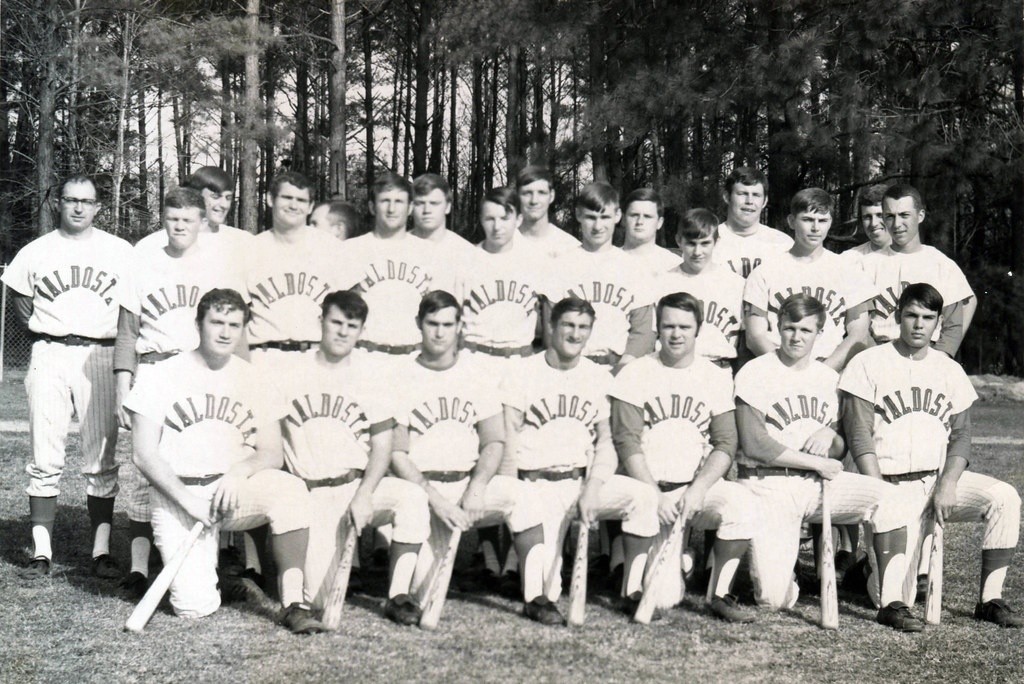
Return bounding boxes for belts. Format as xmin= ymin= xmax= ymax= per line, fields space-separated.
xmin=357 ymin=340 xmax=423 ymax=356
xmin=459 ymin=341 xmax=539 ymax=359
xmin=180 ymin=474 xmax=224 ymax=486
xmin=38 ymin=333 xmax=116 ymax=348
xmin=517 ymin=468 xmax=586 ymax=481
xmin=587 ymin=354 xmax=621 ymax=365
xmin=883 ymin=468 xmax=940 ymax=485
xmin=423 ymin=470 xmax=470 ymax=483
xmin=301 ymin=470 xmax=366 ymax=490
xmin=248 ymin=340 xmax=311 ymax=353
xmin=737 ymin=466 xmax=814 ymax=480
xmin=138 ymin=352 xmax=173 ymax=364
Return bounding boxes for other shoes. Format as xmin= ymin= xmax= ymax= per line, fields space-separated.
xmin=878 ymin=600 xmax=921 ymax=631
xmin=708 ymin=594 xmax=754 ymax=622
xmin=281 ymin=601 xmax=320 ymax=634
xmin=385 ymin=594 xmax=420 ymax=626
xmin=92 ymin=554 xmax=121 ymax=580
xmin=974 ymin=599 xmax=1023 ymax=628
xmin=20 ymin=555 xmax=52 ymax=579
xmin=525 ymin=595 xmax=565 ymax=626
xmin=627 ymin=596 xmax=663 ymax=625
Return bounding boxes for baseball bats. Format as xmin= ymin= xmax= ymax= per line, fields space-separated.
xmin=566 ymin=520 xmax=589 ymax=635
xmin=317 ymin=523 xmax=358 ymax=631
xmin=417 ymin=527 xmax=462 ymax=633
xmin=632 ymin=514 xmax=687 ymax=626
xmin=124 ymin=520 xmax=204 ymax=633
xmin=820 ymin=479 xmax=839 ymax=631
xmin=924 ymin=521 xmax=943 ymax=626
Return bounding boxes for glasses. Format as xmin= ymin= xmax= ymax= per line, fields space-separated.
xmin=59 ymin=196 xmax=99 ymax=206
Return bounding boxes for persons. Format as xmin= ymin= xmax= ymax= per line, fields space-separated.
xmin=274 ymin=290 xmax=429 ymax=628
xmin=123 ymin=289 xmax=322 ymax=632
xmin=2 ymin=165 xmax=1020 ymax=634
